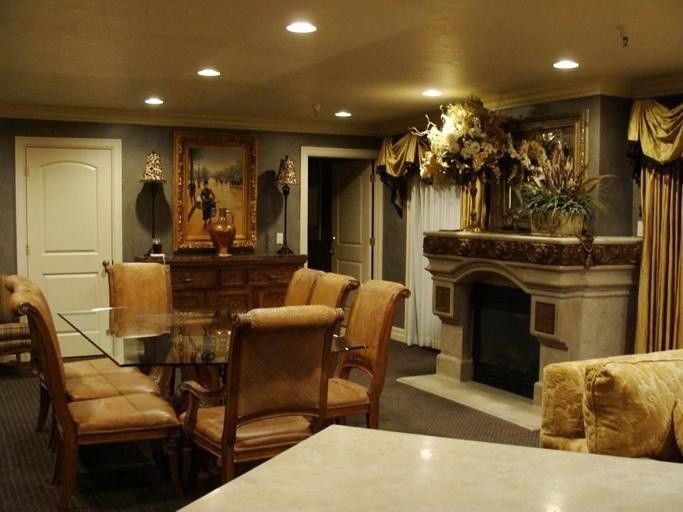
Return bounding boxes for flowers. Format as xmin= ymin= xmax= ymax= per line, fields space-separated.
xmin=507 ymin=133 xmax=618 ymax=242
xmin=408 ymin=94 xmax=510 ymax=186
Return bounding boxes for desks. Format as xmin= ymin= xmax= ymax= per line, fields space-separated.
xmin=175 ymin=424 xmax=683 ymax=512
xmin=56 ymin=309 xmax=371 ymax=409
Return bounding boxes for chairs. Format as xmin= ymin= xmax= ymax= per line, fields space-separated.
xmin=314 ymin=279 xmax=411 ymax=430
xmin=179 ymin=304 xmax=345 ymax=485
xmin=103 ymin=262 xmax=174 ymax=307
xmin=106 ymin=310 xmax=172 ymax=340
xmin=282 ymin=268 xmax=324 ymax=306
xmin=4 ymin=274 xmax=140 ymax=431
xmin=2 ymin=292 xmax=182 ymax=510
xmin=307 ymin=272 xmax=361 ymax=338
xmin=12 ymin=279 xmax=161 ymax=484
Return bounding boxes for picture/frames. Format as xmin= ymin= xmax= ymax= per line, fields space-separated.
xmin=500 ymin=109 xmax=589 ymax=222
xmin=172 ymin=127 xmax=258 ymax=252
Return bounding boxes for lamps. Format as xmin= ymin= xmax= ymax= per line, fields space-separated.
xmin=274 ymin=155 xmax=299 ymax=254
xmin=140 ymin=151 xmax=167 ymax=256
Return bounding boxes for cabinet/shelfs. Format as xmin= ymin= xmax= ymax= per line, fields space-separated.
xmin=171 ymin=266 xmax=300 ymax=312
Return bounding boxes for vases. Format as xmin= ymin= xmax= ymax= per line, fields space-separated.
xmin=529 ymin=209 xmax=584 ymax=238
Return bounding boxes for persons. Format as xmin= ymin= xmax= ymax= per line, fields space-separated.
xmin=188 ymin=173 xmax=243 ymax=227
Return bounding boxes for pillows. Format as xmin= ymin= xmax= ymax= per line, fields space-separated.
xmin=582 ymin=356 xmax=683 ymax=461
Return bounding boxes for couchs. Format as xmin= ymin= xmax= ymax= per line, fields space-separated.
xmin=539 ymin=349 xmax=683 ymax=461
